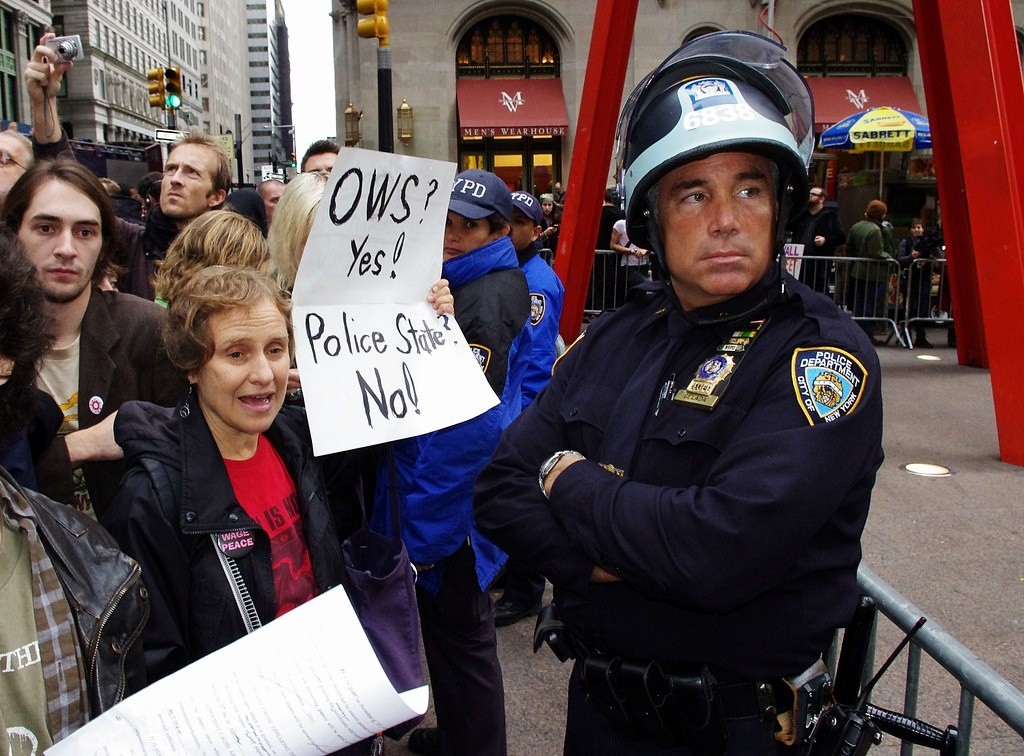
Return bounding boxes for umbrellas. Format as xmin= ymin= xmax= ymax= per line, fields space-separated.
xmin=818 ymin=106 xmax=932 ymax=201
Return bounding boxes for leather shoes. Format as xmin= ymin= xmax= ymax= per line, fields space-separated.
xmin=491 ymin=594 xmax=543 ymax=628
xmin=407 ymin=726 xmax=447 ymax=756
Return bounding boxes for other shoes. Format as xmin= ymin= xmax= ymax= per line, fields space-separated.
xmin=871 ymin=336 xmax=887 ymax=347
xmin=913 ymin=337 xmax=933 ymax=349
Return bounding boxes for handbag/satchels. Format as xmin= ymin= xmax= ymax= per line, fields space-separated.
xmin=340 ymin=523 xmax=425 ymax=693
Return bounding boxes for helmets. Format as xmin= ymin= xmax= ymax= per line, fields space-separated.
xmin=612 ymin=53 xmax=811 ymax=251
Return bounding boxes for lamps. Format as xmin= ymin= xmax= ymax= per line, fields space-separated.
xmin=344 ymin=102 xmax=360 ymax=149
xmin=396 ymin=97 xmax=414 ymax=146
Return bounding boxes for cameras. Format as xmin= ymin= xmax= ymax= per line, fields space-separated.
xmin=45 ymin=35 xmax=85 ymax=63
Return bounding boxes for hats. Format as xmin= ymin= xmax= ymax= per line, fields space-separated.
xmin=224 ymin=187 xmax=268 ymax=237
xmin=510 ymin=190 xmax=544 ymax=225
xmin=449 ymin=168 xmax=513 ymax=222
xmin=539 ymin=193 xmax=555 ymax=203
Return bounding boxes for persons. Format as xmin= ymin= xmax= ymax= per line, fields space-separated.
xmin=470 ymin=30 xmax=885 ymax=756
xmin=584 ymin=189 xmax=657 ymax=324
xmin=0 ymin=33 xmax=565 ymax=756
xmin=788 ymin=188 xmax=955 ymax=347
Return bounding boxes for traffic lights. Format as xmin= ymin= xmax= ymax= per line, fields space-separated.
xmin=147 ymin=67 xmax=166 ymax=110
xmin=290 ymin=153 xmax=296 ymax=168
xmin=357 ymin=0 xmax=389 ymax=40
xmin=165 ymin=66 xmax=182 ymax=111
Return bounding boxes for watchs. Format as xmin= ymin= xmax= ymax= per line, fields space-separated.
xmin=538 ymin=449 xmax=584 ymax=497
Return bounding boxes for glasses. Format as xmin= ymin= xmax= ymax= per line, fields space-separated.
xmin=0 ymin=151 xmax=28 ymax=171
xmin=809 ymin=192 xmax=821 ymax=198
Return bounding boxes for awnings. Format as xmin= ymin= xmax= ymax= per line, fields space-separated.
xmin=803 ymin=75 xmax=922 ymax=133
xmin=457 ymin=78 xmax=568 ymax=137
